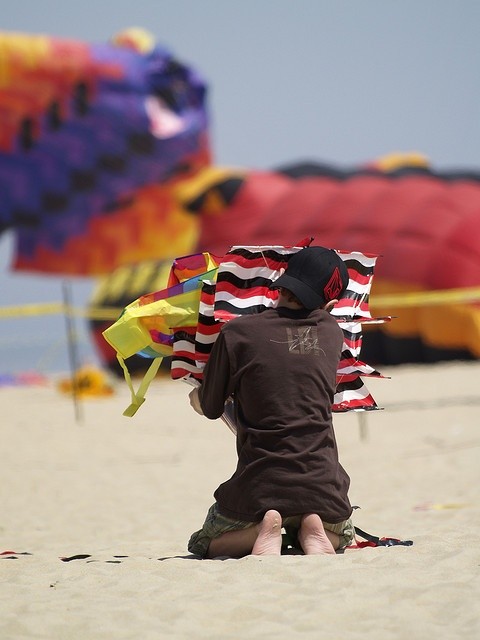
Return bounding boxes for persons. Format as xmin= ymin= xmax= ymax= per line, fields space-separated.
xmin=188 ymin=246 xmax=356 ymax=556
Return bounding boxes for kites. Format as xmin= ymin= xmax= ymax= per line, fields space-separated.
xmin=101 ymin=237 xmax=397 ymax=417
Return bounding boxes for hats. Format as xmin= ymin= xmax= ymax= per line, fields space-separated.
xmin=269 ymin=245 xmax=348 ymax=310
